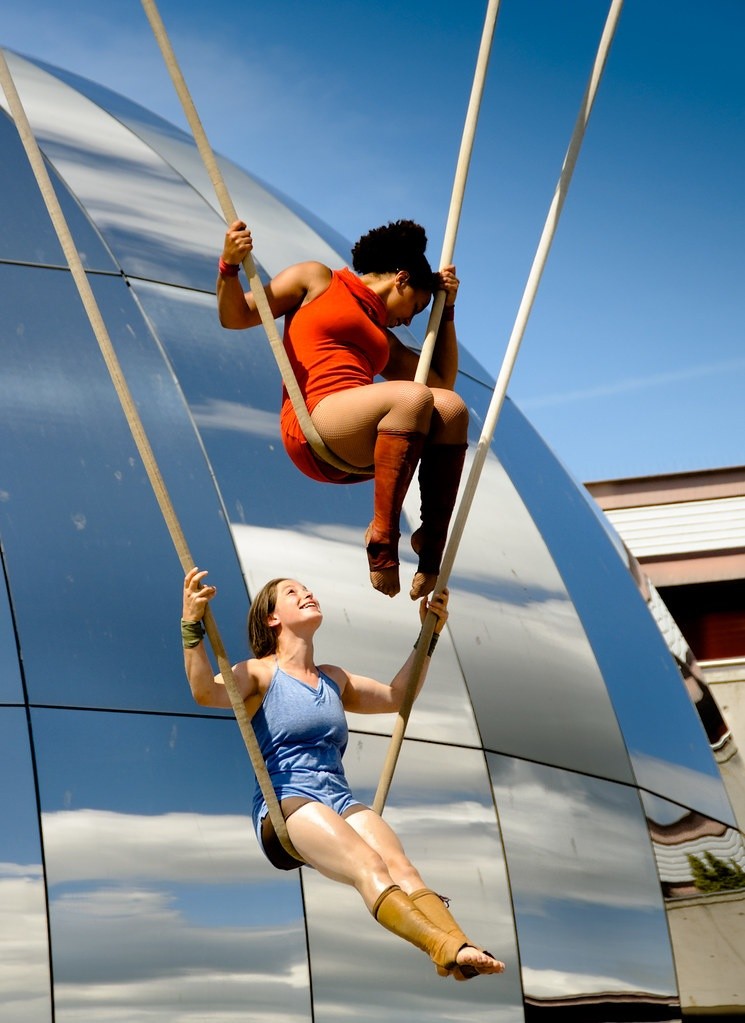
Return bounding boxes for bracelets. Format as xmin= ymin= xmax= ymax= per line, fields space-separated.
xmin=181 ymin=619 xmax=206 ymax=650
xmin=442 ymin=306 xmax=456 ymax=320
xmin=219 ymin=259 xmax=241 ymax=278
xmin=414 ymin=628 xmax=438 ymax=658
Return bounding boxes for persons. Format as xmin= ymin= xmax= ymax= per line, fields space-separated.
xmin=180 ymin=566 xmax=507 ymax=981
xmin=216 ymin=219 xmax=471 ymax=599
